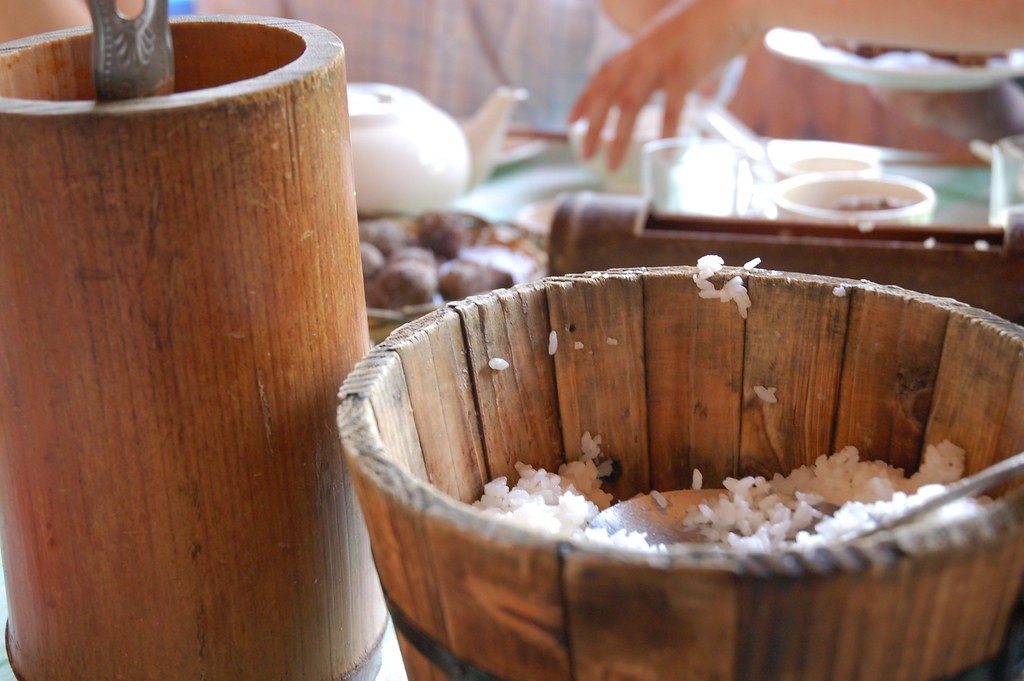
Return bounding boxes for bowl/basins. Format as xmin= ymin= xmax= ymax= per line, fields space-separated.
xmin=571 ymin=107 xmax=706 ymax=189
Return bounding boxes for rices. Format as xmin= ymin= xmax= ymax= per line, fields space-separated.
xmin=462 ymin=255 xmax=1008 ymax=552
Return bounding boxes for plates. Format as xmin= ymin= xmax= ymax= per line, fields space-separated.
xmin=764 ymin=26 xmax=1024 ymax=92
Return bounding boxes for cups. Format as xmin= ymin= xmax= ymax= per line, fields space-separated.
xmin=774 ymin=172 xmax=937 ymax=234
xmin=987 ymin=134 xmax=1024 ymax=229
xmin=641 ymin=136 xmax=883 ymax=224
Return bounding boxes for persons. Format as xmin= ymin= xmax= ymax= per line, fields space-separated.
xmin=567 ymin=0 xmax=1024 ymax=170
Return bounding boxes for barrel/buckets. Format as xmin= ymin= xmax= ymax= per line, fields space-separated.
xmin=334 ymin=265 xmax=1024 ymax=681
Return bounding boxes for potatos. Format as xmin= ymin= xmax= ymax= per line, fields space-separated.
xmin=355 ymin=210 xmax=525 ymax=309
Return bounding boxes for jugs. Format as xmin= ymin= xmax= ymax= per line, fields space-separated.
xmin=346 ymin=76 xmax=526 ymax=216
